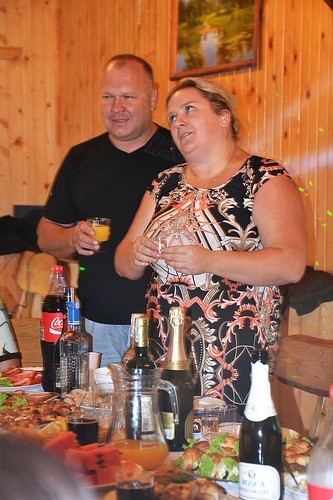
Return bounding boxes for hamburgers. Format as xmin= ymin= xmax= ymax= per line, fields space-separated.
xmin=173 ymin=434 xmax=314 ymax=483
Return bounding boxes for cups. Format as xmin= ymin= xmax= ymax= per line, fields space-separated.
xmin=88 ymin=352 xmax=102 ymax=389
xmin=86 ymin=217 xmax=111 ymax=255
xmin=117 ymin=471 xmax=155 ymax=500
xmin=64 ymin=410 xmax=98 ymax=445
xmin=153 ymin=237 xmax=168 ymax=259
xmin=202 ymin=403 xmax=238 ymax=442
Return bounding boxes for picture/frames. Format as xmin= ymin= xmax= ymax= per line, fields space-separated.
xmin=168 ymin=0 xmax=262 ymax=82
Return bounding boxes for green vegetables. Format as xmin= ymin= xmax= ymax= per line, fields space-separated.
xmin=0 ymin=372 xmax=28 ymax=413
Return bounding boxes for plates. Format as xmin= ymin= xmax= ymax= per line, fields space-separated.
xmin=0 ymin=366 xmax=123 ymax=430
xmin=83 ymin=460 xmax=143 ymax=491
xmin=170 ymin=450 xmax=239 ymax=488
xmin=217 ymin=424 xmax=300 ymax=442
xmin=192 ymin=395 xmax=226 ymax=411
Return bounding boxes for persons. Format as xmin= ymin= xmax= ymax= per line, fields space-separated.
xmin=0 ymin=295 xmax=24 ymax=372
xmin=113 ymin=76 xmax=305 ymax=417
xmin=35 ymin=54 xmax=187 ymax=368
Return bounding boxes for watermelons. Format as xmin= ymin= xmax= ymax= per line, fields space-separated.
xmin=41 ymin=430 xmax=135 ymax=486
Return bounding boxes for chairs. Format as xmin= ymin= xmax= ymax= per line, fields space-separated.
xmin=274 ymin=266 xmax=333 ymax=440
xmin=8 ymin=248 xmax=59 ymax=368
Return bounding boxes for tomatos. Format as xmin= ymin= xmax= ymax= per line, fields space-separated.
xmin=4 ymin=367 xmax=42 ymax=387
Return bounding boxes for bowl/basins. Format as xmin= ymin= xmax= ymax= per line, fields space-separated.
xmin=92 ymin=367 xmax=114 ymax=392
xmin=285 ymin=474 xmax=308 ymax=500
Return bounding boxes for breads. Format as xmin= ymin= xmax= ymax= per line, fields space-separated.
xmin=100 ymin=468 xmax=231 ymax=500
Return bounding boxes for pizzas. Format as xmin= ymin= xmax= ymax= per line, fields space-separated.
xmin=0 ymin=399 xmax=80 ymax=432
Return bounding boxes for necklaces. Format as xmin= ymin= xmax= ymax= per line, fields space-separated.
xmin=190 ymin=148 xmax=234 ymax=181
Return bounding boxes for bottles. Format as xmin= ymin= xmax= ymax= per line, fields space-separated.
xmin=125 ymin=317 xmax=157 ymax=440
xmin=306 ymin=383 xmax=333 ymax=500
xmin=159 ymin=306 xmax=194 ymax=453
xmin=239 ymin=348 xmax=284 ymax=500
xmin=40 ymin=265 xmax=89 ymax=400
xmin=120 ymin=313 xmax=155 ymax=369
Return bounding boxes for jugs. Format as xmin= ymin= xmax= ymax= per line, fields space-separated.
xmin=104 ymin=362 xmax=180 ymax=471
